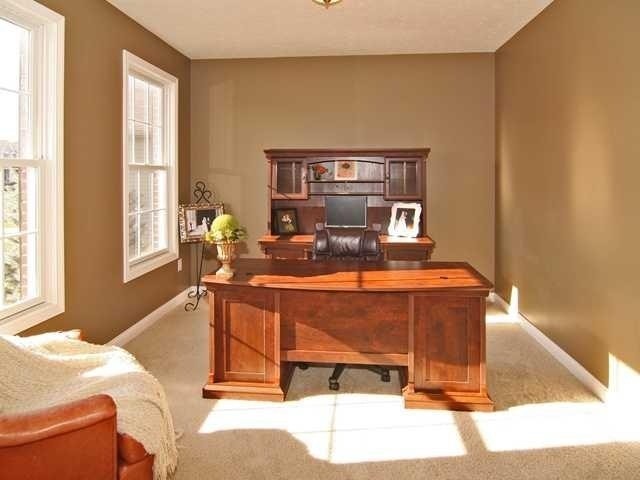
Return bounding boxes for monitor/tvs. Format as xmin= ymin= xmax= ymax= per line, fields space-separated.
xmin=325 ymin=195 xmax=367 ymax=228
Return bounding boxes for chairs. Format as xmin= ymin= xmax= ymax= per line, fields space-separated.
xmin=0 ymin=332 xmax=180 ymax=479
xmin=298 ymin=228 xmax=389 ymax=391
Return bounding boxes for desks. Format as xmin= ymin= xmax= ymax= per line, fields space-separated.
xmin=200 ymin=258 xmax=496 ymax=412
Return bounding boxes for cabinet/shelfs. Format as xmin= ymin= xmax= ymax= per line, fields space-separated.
xmin=265 ymin=148 xmax=436 ymax=263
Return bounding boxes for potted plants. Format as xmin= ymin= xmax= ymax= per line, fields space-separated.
xmin=206 ymin=214 xmax=248 ymax=281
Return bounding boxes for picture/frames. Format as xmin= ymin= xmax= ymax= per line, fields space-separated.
xmin=179 ymin=204 xmax=223 ymax=243
xmin=389 ymin=203 xmax=422 ymax=237
xmin=335 ymin=161 xmax=358 ymax=180
xmin=271 ymin=208 xmax=299 ymax=235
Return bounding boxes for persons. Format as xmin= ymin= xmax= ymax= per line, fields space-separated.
xmin=397 ymin=211 xmax=413 ymax=232
xmin=197 ymin=216 xmax=212 ymax=232
xmin=282 ymin=213 xmax=296 ymax=232
